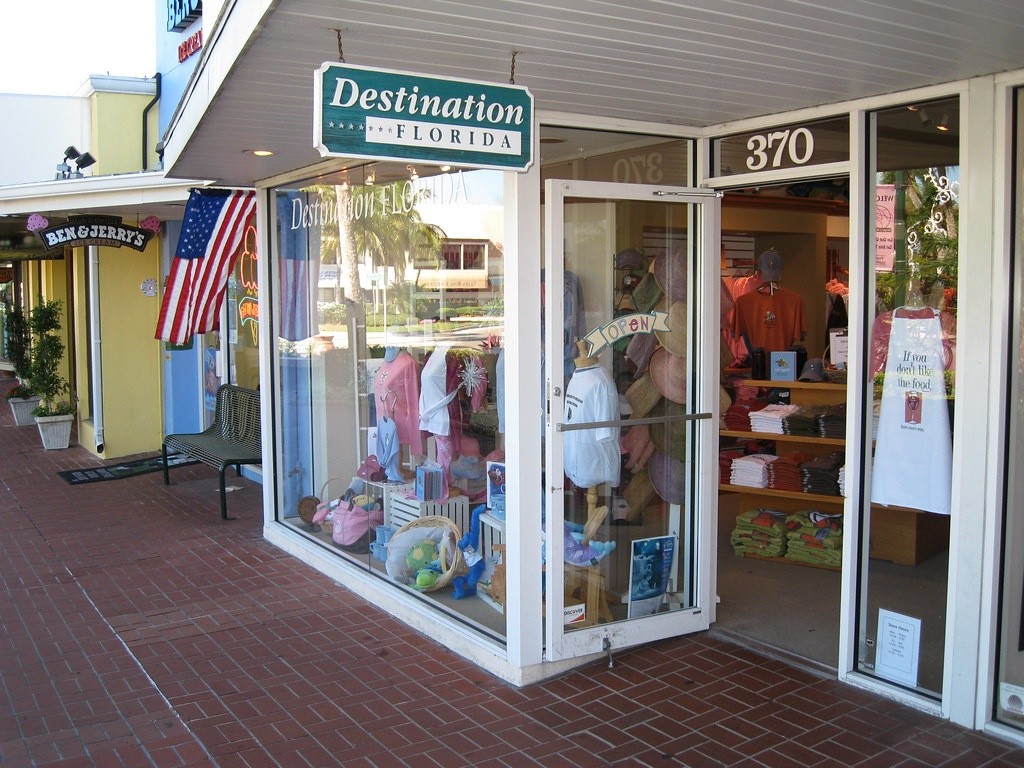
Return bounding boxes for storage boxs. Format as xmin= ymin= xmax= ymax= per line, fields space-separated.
xmin=829 ymin=328 xmax=848 ymax=365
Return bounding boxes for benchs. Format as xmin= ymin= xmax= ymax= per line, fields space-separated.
xmin=162 ymin=384 xmax=262 ymax=521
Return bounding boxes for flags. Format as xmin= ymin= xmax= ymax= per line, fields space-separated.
xmin=154 ymin=188 xmax=258 ymax=346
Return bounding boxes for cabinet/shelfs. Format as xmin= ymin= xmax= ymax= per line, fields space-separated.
xmin=719 ymin=379 xmax=955 ymax=571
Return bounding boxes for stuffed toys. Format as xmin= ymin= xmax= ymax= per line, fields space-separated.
xmin=452 ymin=505 xmax=486 ymax=599
xmin=406 ymin=539 xmax=450 ymax=588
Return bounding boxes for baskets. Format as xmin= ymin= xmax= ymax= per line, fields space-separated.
xmin=318 ymin=478 xmax=348 ymax=536
xmin=822 ymin=345 xmax=847 ymax=383
xmin=385 ymin=516 xmax=463 ymax=592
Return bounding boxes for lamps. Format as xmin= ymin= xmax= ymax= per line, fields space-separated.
xmin=406 ymin=164 xmax=420 ymax=181
xmin=364 ymin=169 xmax=376 ymax=185
xmin=69 ymin=152 xmax=96 ymax=178
xmin=56 ymin=146 xmax=81 ymax=179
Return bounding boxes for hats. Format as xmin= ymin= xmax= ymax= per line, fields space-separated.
xmin=798 ymin=358 xmax=829 ymax=381
xmin=758 ymin=250 xmax=783 ymax=281
xmin=449 ymin=454 xmax=483 ymax=479
xmin=616 ymin=248 xmax=687 ymax=503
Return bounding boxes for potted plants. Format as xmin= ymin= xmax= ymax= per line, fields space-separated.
xmin=27 ymin=291 xmax=75 ymax=449
xmin=0 ymin=281 xmax=43 ymax=427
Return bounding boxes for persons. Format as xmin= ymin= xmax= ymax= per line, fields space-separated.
xmin=563 ymin=353 xmax=621 ymax=489
xmin=374 ymin=345 xmax=424 ymax=456
xmin=418 ymin=339 xmax=463 ymax=440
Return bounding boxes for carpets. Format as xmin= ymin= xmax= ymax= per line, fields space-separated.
xmin=57 ymin=451 xmax=201 ymax=485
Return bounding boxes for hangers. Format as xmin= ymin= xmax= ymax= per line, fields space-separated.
xmin=825 ymin=267 xmax=849 ymax=288
xmin=757 ymin=284 xmax=778 ymax=292
xmin=731 ymin=260 xmax=761 ymax=277
